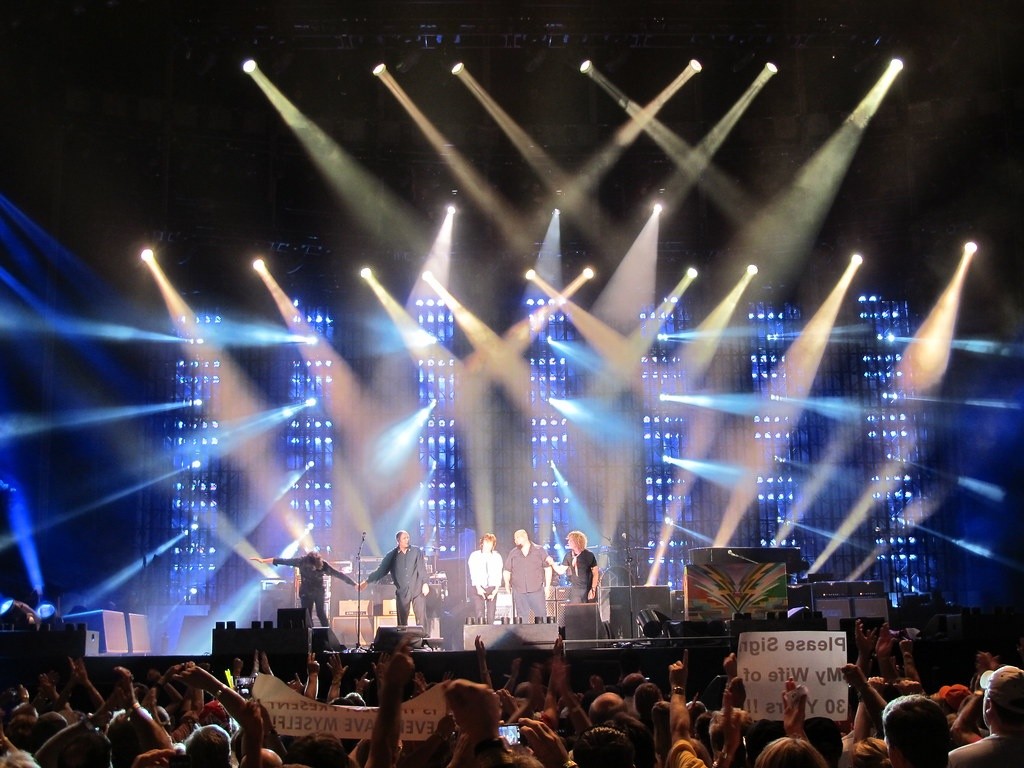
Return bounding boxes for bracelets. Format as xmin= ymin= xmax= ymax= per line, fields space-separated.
xmin=592 ymin=586 xmax=597 ymax=598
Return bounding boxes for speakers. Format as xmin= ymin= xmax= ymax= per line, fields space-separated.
xmin=632 ymin=586 xmax=670 ymax=638
xmin=597 ymin=586 xmax=632 ymax=640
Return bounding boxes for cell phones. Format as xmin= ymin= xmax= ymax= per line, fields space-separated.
xmin=235 ymin=676 xmax=256 ymax=689
xmin=498 ymin=723 xmax=527 ymax=748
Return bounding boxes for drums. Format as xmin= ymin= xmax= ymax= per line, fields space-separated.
xmin=600 ymin=566 xmax=634 ymax=586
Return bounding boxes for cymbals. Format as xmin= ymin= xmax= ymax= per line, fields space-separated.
xmin=625 ymin=547 xmax=651 ymax=550
xmin=587 ymin=546 xmax=612 ymax=549
xmin=598 ymin=550 xmax=618 ymax=553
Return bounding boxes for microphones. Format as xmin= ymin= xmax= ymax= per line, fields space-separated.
xmin=358 ymin=531 xmax=367 ymax=555
xmin=622 ymin=532 xmax=628 ymax=540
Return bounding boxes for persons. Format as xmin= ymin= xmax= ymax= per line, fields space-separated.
xmin=548 ymin=530 xmax=600 ymax=604
xmin=0 ymin=615 xmax=1024 ymax=768
xmin=469 ymin=533 xmax=504 ymax=623
xmin=503 ymin=527 xmax=554 ymax=624
xmin=357 ymin=530 xmax=431 ymax=638
xmin=248 ymin=551 xmax=360 ymax=628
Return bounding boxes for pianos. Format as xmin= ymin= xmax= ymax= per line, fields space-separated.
xmin=687 ymin=547 xmax=808 ymax=585
